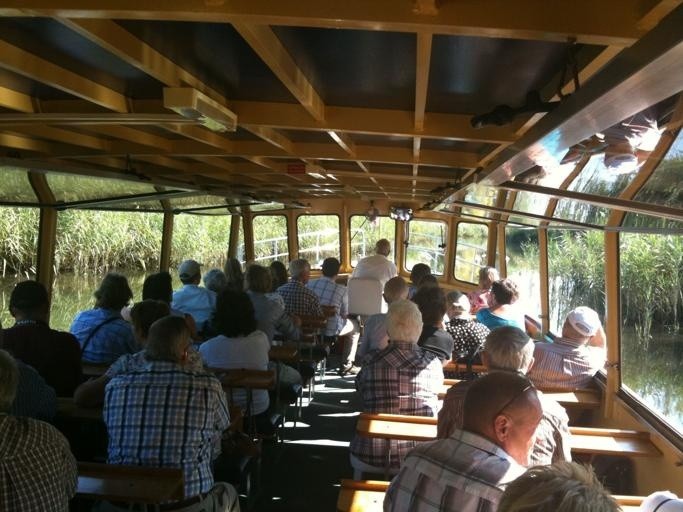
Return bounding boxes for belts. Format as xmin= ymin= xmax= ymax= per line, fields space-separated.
xmin=110 ymin=494 xmax=207 ymax=512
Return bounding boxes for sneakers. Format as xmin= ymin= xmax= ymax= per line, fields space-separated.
xmin=340 ymin=363 xmax=361 ymax=375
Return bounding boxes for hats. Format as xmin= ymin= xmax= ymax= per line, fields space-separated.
xmin=179 ymin=260 xmax=204 ymax=280
xmin=567 ymin=306 xmax=599 ymax=336
xmin=12 ymin=281 xmax=47 ymax=308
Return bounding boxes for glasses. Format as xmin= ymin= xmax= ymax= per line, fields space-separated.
xmin=493 ymin=379 xmax=536 ymax=434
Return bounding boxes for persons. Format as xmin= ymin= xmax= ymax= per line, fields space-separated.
xmin=0 ymin=240 xmax=622 ymax=511
